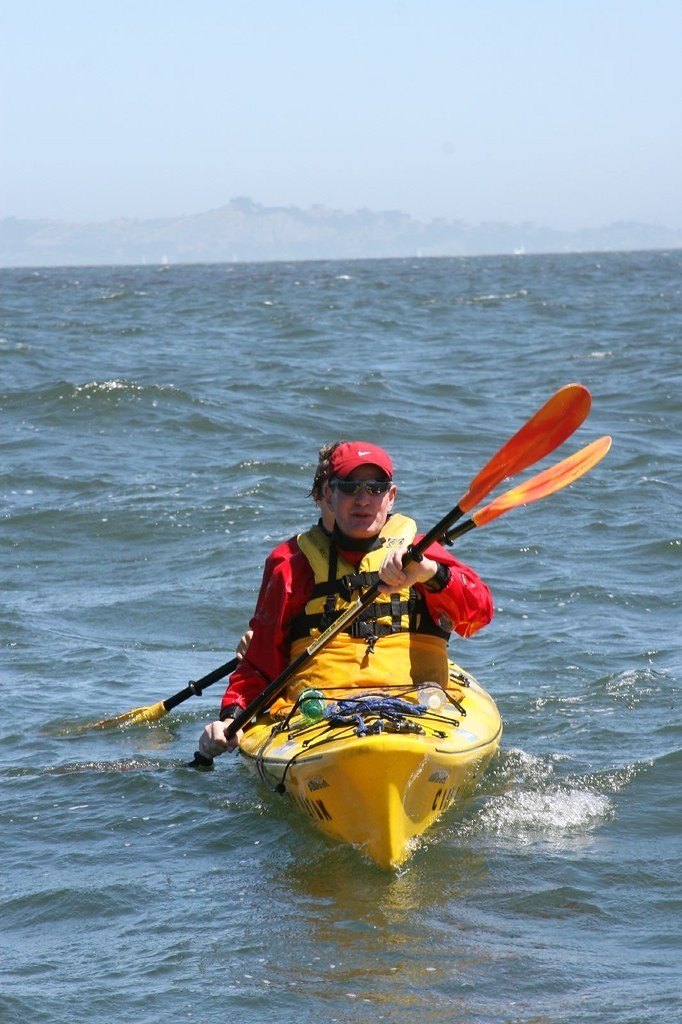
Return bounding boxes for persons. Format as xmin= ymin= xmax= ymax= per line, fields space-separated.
xmin=198 ymin=439 xmax=495 ymax=759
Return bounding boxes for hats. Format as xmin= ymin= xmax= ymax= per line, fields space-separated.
xmin=329 ymin=440 xmax=392 ymax=480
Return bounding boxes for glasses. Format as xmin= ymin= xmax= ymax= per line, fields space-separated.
xmin=330 ymin=478 xmax=388 ymax=496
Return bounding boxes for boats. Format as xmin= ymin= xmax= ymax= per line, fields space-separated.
xmin=236 ymin=655 xmax=504 ymax=872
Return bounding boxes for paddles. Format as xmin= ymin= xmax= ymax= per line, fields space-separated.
xmin=174 ymin=381 xmax=593 ymax=772
xmin=92 ymin=433 xmax=614 ymax=727
